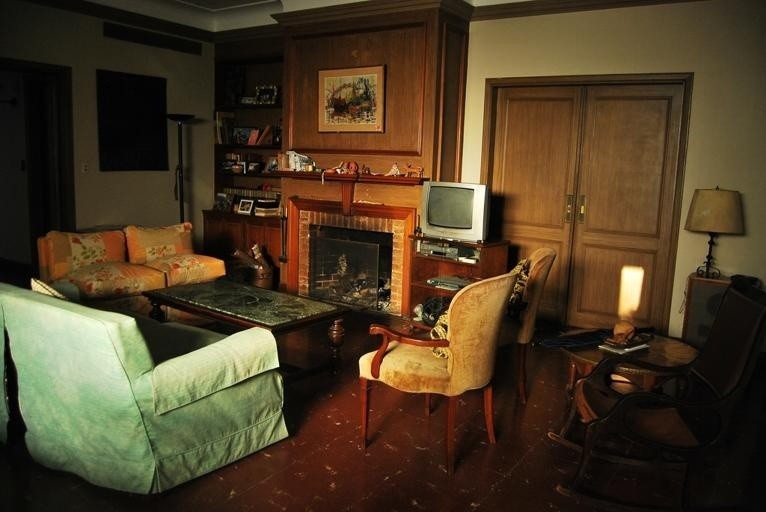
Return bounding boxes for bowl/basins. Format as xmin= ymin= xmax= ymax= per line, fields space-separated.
xmin=231 ymin=164 xmax=243 ymax=175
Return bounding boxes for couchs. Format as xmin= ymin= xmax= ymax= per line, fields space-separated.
xmin=37 ymin=225 xmax=216 ymax=326
xmin=0 ymin=283 xmax=290 ymax=495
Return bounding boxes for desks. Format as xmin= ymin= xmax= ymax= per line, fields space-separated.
xmin=558 ymin=331 xmax=702 ymax=422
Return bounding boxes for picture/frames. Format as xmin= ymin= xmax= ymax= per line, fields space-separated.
xmin=238 ymin=198 xmax=255 ymax=216
xmin=254 ymin=86 xmax=277 ymax=105
xmin=231 ymin=127 xmax=259 ymax=145
xmin=315 ymin=64 xmax=387 ymax=134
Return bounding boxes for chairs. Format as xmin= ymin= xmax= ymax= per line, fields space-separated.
xmin=357 ymin=264 xmax=518 ymax=476
xmin=547 ymin=275 xmax=766 ymax=511
xmin=438 ymin=247 xmax=556 ymax=405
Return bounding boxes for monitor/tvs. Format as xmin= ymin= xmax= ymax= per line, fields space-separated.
xmin=421 ymin=181 xmax=491 ymax=244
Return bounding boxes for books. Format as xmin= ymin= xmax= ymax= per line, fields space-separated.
xmin=216 ymin=110 xmax=274 ymax=145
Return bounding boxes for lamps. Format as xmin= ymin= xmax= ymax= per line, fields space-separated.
xmin=684 ymin=188 xmax=747 ymax=279
xmin=614 ymin=266 xmax=645 ymax=340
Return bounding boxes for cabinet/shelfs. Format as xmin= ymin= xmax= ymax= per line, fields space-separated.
xmin=214 ymin=57 xmax=282 ymax=205
xmin=681 ymin=272 xmax=731 ymax=341
xmin=401 ymin=234 xmax=510 ymax=331
xmin=201 ymin=210 xmax=280 ymax=291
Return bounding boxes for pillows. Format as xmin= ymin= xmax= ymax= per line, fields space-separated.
xmin=124 ymin=222 xmax=194 ymax=264
xmin=65 ymin=262 xmax=166 ymax=299
xmin=145 ymin=254 xmax=225 ymax=287
xmin=45 ymin=230 xmax=125 ymax=281
